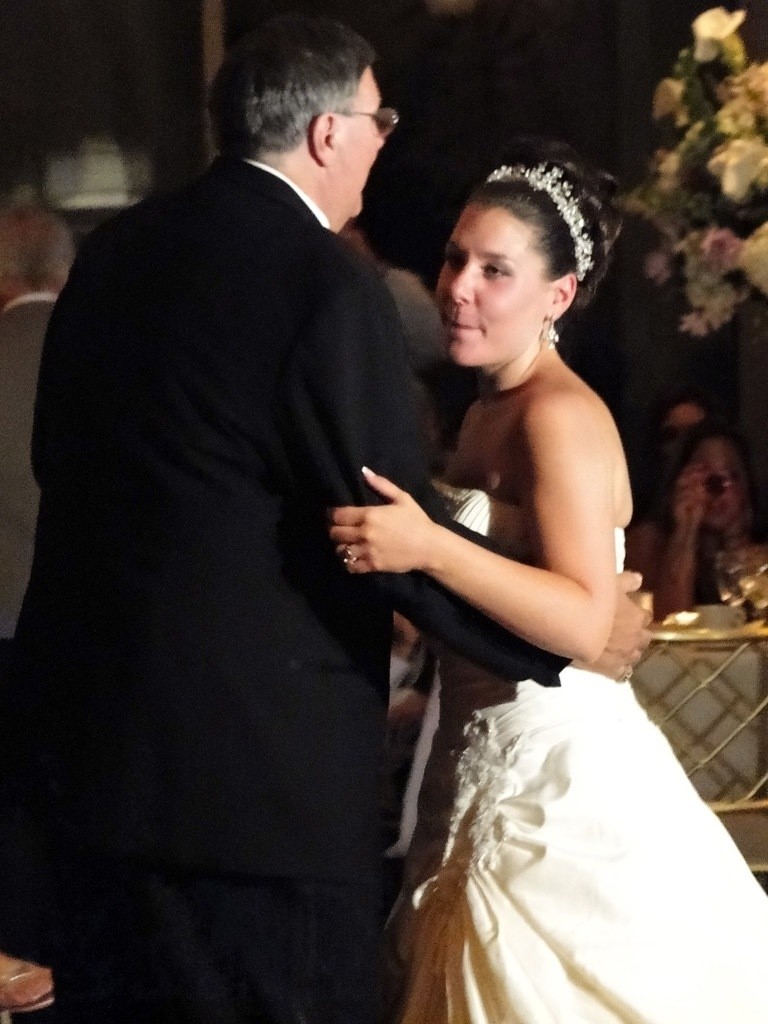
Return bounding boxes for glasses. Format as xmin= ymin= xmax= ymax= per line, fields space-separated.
xmin=334 ymin=106 xmax=399 ymax=139
xmin=706 ymin=470 xmax=740 ymax=497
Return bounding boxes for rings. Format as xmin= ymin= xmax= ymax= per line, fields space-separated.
xmin=343 ymin=542 xmax=356 ymax=565
xmin=617 ymin=664 xmax=633 ymax=682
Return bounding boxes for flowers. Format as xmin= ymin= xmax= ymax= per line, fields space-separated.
xmin=614 ymin=5 xmax=768 ymax=338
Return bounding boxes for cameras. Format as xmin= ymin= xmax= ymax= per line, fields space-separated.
xmin=704 ymin=471 xmax=733 ymax=497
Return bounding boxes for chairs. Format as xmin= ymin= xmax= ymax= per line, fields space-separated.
xmin=631 ymin=625 xmax=768 ymax=884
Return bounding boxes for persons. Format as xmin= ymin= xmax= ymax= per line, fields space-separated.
xmin=0 ymin=18 xmax=768 ymax=1024
